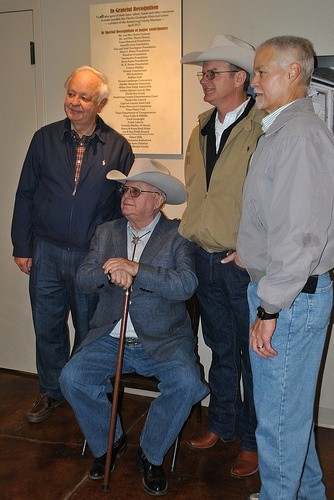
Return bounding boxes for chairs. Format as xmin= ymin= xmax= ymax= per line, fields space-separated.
xmin=80 ymin=290 xmax=204 ymax=472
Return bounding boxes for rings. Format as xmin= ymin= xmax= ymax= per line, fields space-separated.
xmin=258 ymin=345 xmax=264 ymax=349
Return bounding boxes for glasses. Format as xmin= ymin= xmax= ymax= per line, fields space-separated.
xmin=121 ymin=185 xmax=160 ymax=198
xmin=196 ymin=69 xmax=238 ymax=81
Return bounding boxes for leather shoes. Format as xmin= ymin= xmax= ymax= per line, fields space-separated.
xmin=185 ymin=432 xmax=235 ymax=450
xmin=89 ymin=432 xmax=128 ymax=481
xmin=230 ymin=451 xmax=259 ymax=478
xmin=137 ymin=445 xmax=169 ymax=496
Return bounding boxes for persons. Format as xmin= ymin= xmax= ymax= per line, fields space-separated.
xmin=179 ymin=33 xmax=269 ymax=478
xmin=58 ymin=156 xmax=212 ymax=496
xmin=232 ymin=33 xmax=334 ymax=500
xmin=10 ymin=64 xmax=134 ymax=424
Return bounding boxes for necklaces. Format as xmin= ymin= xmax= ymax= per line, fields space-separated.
xmin=127 ymin=229 xmax=150 ymax=245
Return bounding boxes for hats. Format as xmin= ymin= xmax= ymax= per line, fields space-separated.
xmin=180 ymin=34 xmax=256 ymax=83
xmin=106 ymin=158 xmax=189 ymax=205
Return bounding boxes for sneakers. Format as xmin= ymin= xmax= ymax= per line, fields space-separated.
xmin=26 ymin=395 xmax=61 ymax=423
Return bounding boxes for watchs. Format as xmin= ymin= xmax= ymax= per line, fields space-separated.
xmin=255 ymin=305 xmax=279 ymax=320
